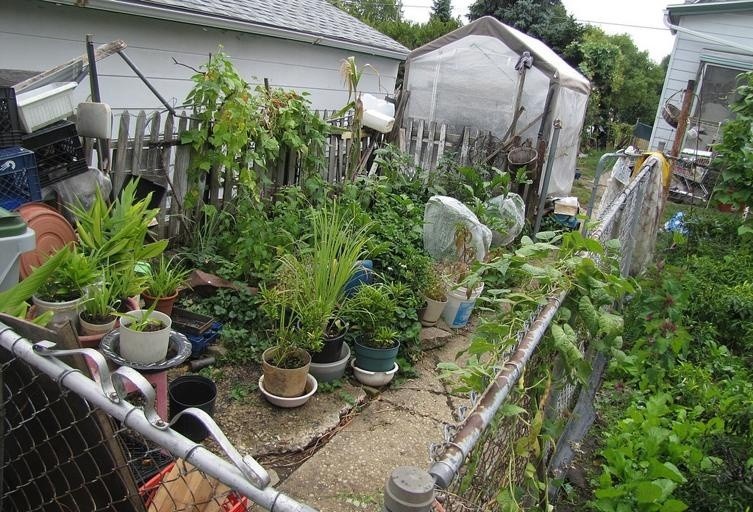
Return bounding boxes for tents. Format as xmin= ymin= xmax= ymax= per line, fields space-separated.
xmin=400 ymin=15 xmax=592 ymax=198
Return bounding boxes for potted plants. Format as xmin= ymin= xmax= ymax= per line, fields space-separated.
xmin=338 ymin=251 xmax=450 ymax=347
xmin=342 ymin=286 xmax=400 ymax=388
xmin=274 ymin=195 xmax=395 ymax=381
xmin=24 ymin=172 xmax=195 ymax=365
xmin=258 ymin=261 xmax=333 ymax=406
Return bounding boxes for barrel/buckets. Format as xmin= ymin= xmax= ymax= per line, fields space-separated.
xmin=340 ymin=259 xmax=373 ymax=296
xmin=508 ymin=146 xmax=538 ymax=183
xmin=442 ymin=265 xmax=484 ymax=329
xmin=33 ymin=291 xmax=85 ymax=325
